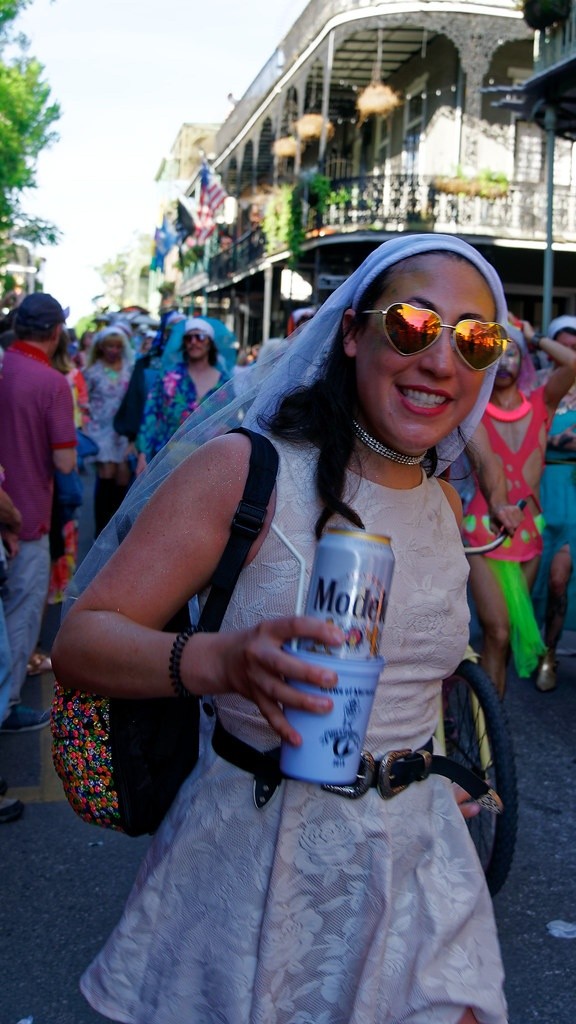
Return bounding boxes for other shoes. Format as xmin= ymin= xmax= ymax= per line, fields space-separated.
xmin=537 ymin=658 xmax=561 ymax=692
xmin=0 ymin=704 xmax=52 ymax=732
xmin=0 ymin=795 xmax=24 ymax=824
xmin=26 ymin=651 xmax=52 ymax=676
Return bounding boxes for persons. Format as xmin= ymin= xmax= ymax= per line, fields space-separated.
xmin=388 ymin=322 xmax=436 ymax=349
xmin=52 ymin=234 xmax=507 ymax=1024
xmin=457 ymin=333 xmax=499 ymax=365
xmin=442 ymin=317 xmax=576 ymax=752
xmin=0 ymin=294 xmax=239 ymax=822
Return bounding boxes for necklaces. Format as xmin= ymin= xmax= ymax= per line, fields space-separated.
xmin=352 ymin=422 xmax=427 ymax=466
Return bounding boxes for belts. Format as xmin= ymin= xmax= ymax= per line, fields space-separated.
xmin=211 ymin=720 xmax=491 ymax=809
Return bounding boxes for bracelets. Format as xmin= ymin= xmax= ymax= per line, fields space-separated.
xmin=169 ymin=625 xmax=211 ymax=700
xmin=531 ymin=332 xmax=546 ymax=350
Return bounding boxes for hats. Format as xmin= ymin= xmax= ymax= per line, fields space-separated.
xmin=185 ymin=318 xmax=215 ymax=339
xmin=507 ymin=322 xmax=527 ymax=355
xmin=16 ymin=294 xmax=71 ymax=330
xmin=549 ymin=315 xmax=576 ymax=339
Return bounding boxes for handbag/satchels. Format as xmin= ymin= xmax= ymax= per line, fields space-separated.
xmin=52 ymin=427 xmax=280 ymax=837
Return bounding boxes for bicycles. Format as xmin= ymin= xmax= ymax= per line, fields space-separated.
xmin=433 ymin=643 xmax=519 ymax=895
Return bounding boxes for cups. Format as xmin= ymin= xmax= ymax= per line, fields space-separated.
xmin=279 ymin=639 xmax=385 ymax=785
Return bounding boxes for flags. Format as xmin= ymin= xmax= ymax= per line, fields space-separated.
xmin=199 ymin=164 xmax=227 ymax=239
xmin=151 ymin=200 xmax=195 ymax=272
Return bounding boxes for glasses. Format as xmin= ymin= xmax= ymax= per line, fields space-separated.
xmin=183 ymin=334 xmax=208 ymax=342
xmin=359 ymin=304 xmax=513 ymax=371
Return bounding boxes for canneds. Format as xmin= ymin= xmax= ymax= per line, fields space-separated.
xmin=297 ymin=524 xmax=395 ymax=661
